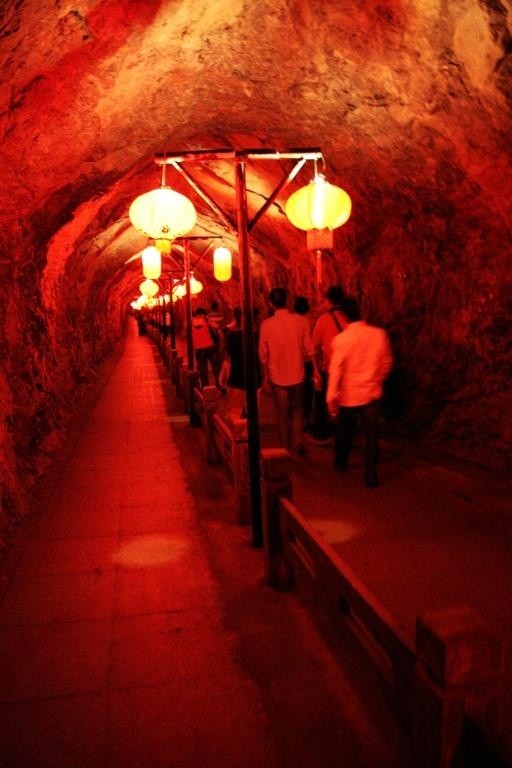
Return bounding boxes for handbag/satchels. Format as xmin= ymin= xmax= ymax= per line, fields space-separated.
xmin=219 ymin=360 xmax=231 ymax=388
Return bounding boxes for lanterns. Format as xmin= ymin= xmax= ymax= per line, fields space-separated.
xmin=141 ymin=246 xmax=163 ymax=280
xmin=128 ymin=184 xmax=198 ymax=255
xmin=285 ymin=174 xmax=352 ymax=285
xmin=130 ymin=275 xmax=204 ymax=311
xmin=213 ymin=246 xmax=233 ymax=282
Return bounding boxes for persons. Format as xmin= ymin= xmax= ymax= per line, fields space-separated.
xmin=187 ymin=300 xmax=276 ymax=442
xmin=257 ymin=287 xmax=324 ymax=458
xmin=325 ymin=297 xmax=395 ymax=489
xmin=135 ymin=307 xmax=172 ymax=339
xmin=305 ymin=286 xmax=350 ymax=446
xmin=290 ymin=295 xmax=316 ymax=423
